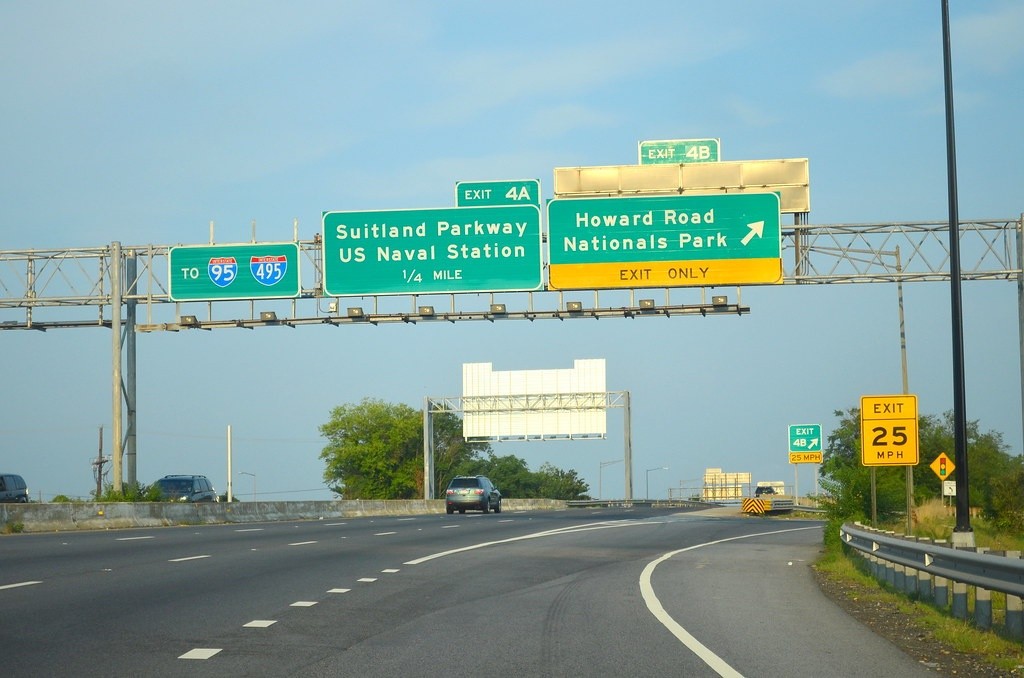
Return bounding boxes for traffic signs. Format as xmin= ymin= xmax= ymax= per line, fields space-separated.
xmin=547 ymin=191 xmax=783 ymax=290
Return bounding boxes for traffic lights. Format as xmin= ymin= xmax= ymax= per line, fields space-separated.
xmin=940 ymin=458 xmax=946 ymax=475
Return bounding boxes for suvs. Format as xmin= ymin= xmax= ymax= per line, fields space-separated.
xmin=446 ymin=475 xmax=502 ymax=514
xmin=153 ymin=474 xmax=219 ymax=503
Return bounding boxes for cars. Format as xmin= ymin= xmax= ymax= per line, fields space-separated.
xmin=0 ymin=473 xmax=29 ymax=504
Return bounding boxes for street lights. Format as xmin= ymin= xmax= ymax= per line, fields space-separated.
xmin=781 ymin=245 xmax=917 ymax=526
xmin=238 ymin=472 xmax=256 ymax=502
xmin=646 ymin=467 xmax=669 ymax=500
